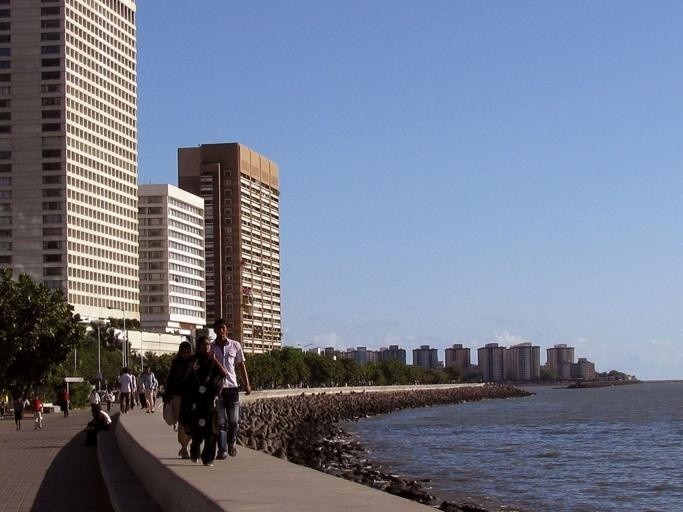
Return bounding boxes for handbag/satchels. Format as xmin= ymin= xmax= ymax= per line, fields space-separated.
xmin=163 ymin=395 xmax=181 ymax=425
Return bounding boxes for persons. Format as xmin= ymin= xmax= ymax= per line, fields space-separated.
xmin=0 ymin=401 xmax=4 ymax=414
xmin=31 ymin=396 xmax=43 ymax=430
xmin=61 ymin=389 xmax=69 ymax=417
xmin=177 ymin=335 xmax=228 ymax=467
xmin=88 ymin=365 xmax=163 ymax=430
xmin=206 ymin=318 xmax=251 ymax=461
xmin=12 ymin=398 xmax=22 ymax=430
xmin=19 ymin=395 xmax=30 ymax=417
xmin=164 ymin=341 xmax=194 ymax=458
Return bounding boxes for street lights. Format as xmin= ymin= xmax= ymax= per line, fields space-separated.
xmin=73 ymin=305 xmax=133 ymax=394
xmin=295 ymin=341 xmax=313 ymax=355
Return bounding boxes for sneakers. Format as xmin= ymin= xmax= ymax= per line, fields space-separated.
xmin=178 ymin=444 xmax=236 ymax=466
